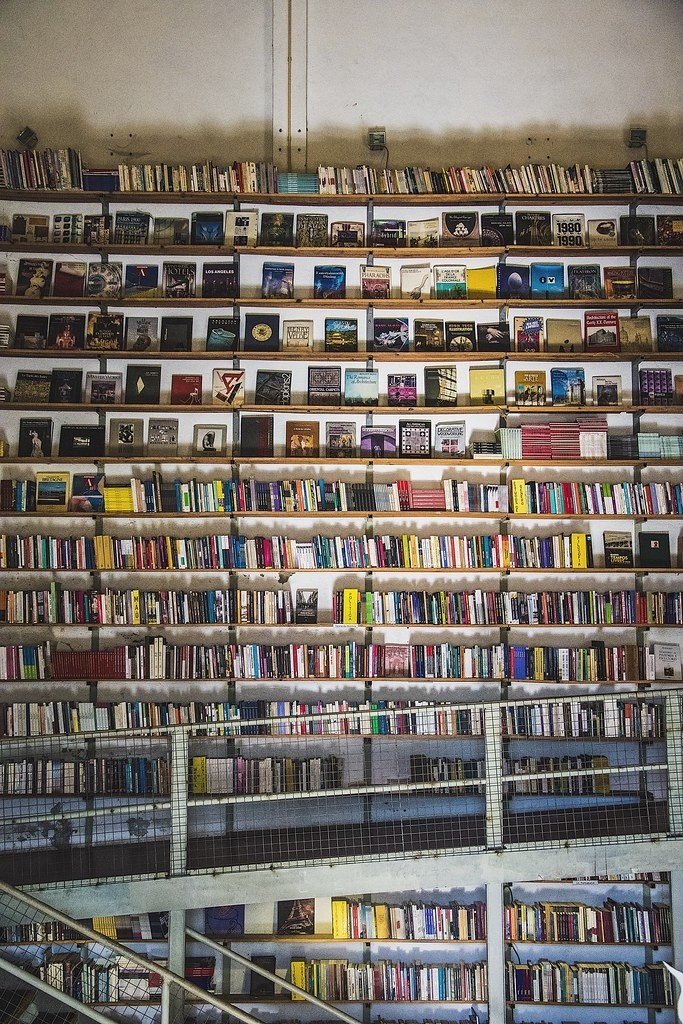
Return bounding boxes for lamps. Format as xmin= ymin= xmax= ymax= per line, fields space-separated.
xmin=17 ymin=126 xmax=38 ymax=147
xmin=628 ymin=128 xmax=647 ymax=146
xmin=369 ymin=131 xmax=387 ymax=148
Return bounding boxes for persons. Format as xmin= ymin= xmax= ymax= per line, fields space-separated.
xmin=204 ymin=433 xmax=213 ymax=447
xmin=480 ymin=326 xmax=509 ymax=343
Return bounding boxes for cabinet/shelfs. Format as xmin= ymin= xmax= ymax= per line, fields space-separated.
xmin=0 ymin=193 xmax=683 ymax=1024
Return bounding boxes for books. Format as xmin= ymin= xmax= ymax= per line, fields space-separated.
xmin=0 ymin=870 xmax=673 ymax=1006
xmin=0 ymin=147 xmax=683 ymax=795
